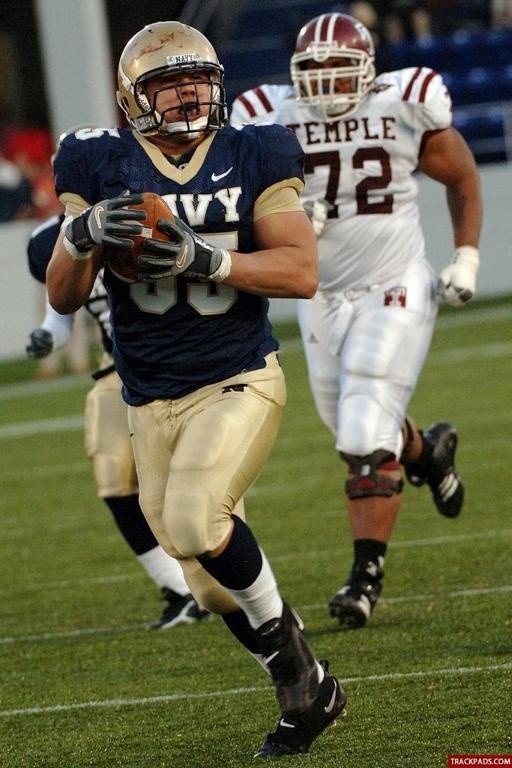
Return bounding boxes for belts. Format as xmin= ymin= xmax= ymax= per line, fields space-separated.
xmin=90 ymin=364 xmax=115 ymax=381
xmin=243 ymin=354 xmax=281 ymax=373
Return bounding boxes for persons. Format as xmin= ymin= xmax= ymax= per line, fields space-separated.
xmin=24 ymin=206 xmax=213 ymax=636
xmin=44 ymin=20 xmax=350 ymax=761
xmin=1 ymin=2 xmax=512 ymax=229
xmin=227 ymin=10 xmax=488 ymax=635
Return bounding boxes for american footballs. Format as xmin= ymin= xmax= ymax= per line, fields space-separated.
xmin=107 ymin=193 xmax=175 ymax=284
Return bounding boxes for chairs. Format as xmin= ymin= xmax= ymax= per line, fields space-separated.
xmin=222 ymin=19 xmax=511 ymax=165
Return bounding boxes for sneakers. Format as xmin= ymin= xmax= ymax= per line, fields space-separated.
xmin=328 ymin=555 xmax=385 ymax=629
xmin=146 ymin=586 xmax=213 ymax=630
xmin=426 ymin=422 xmax=465 ymax=518
xmin=253 ymin=597 xmax=328 ymax=718
xmin=252 ymin=659 xmax=348 ymax=761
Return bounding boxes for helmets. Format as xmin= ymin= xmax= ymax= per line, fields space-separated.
xmin=117 ymin=20 xmax=225 ymax=138
xmin=293 ymin=12 xmax=376 ymax=93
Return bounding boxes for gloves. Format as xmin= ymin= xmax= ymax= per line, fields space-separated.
xmin=135 ymin=214 xmax=224 ymax=286
xmin=64 ymin=190 xmax=148 ymax=254
xmin=437 ymin=245 xmax=480 ymax=309
